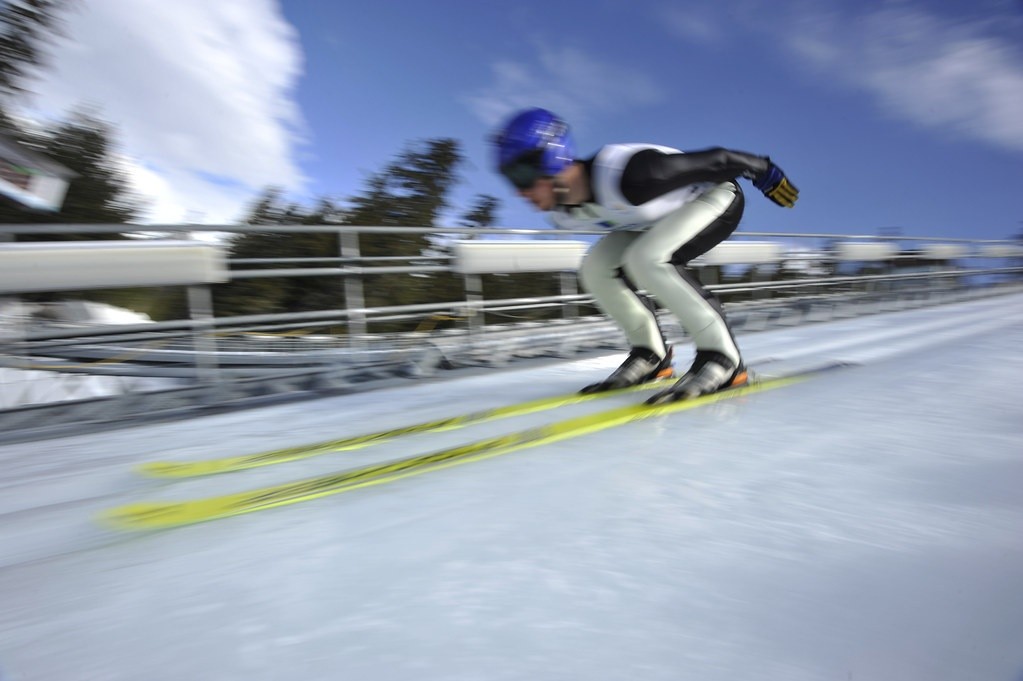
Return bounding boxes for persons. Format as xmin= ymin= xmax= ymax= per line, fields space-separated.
xmin=496 ymin=107 xmax=799 ymax=406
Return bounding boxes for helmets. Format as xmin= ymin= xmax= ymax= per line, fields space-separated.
xmin=494 ymin=108 xmax=576 ymax=192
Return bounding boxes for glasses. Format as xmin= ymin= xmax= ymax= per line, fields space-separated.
xmin=506 ymin=163 xmax=540 ymax=188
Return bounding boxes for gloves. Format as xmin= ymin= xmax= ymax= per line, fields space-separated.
xmin=752 ymin=157 xmax=799 ymax=210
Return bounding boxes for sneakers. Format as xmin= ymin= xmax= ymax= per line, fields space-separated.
xmin=607 ymin=351 xmax=672 ymax=390
xmin=674 ymin=355 xmax=750 ymax=400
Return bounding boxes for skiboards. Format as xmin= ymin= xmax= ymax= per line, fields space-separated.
xmin=99 ymin=356 xmax=863 ymax=532
